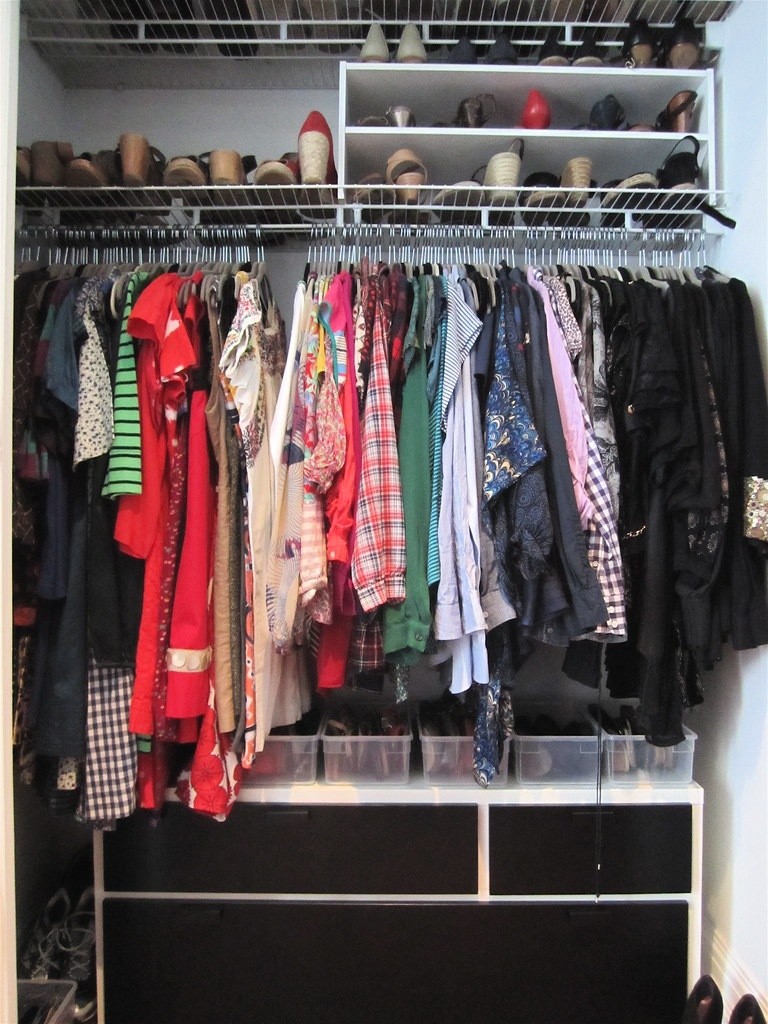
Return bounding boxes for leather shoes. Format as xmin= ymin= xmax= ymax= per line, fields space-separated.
xmin=359 ymin=22 xmax=428 ymax=64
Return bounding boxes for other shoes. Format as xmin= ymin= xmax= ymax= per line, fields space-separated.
xmin=444 ymin=31 xmax=518 ymax=64
xmin=84 ymin=0 xmax=356 ymax=58
xmin=535 ymin=37 xmax=605 ymax=70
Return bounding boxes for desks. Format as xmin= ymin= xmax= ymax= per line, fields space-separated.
xmin=93 ymin=781 xmax=706 ymax=1024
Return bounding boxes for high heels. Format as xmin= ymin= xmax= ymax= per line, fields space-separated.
xmin=681 ymin=975 xmax=767 ymax=1024
xmin=16 ymin=100 xmax=338 ymax=225
xmin=624 ymin=14 xmax=703 ymax=71
xmin=349 ymin=78 xmax=704 ymax=228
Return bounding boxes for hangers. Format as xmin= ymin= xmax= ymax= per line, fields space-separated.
xmin=15 ymin=224 xmax=730 ymax=292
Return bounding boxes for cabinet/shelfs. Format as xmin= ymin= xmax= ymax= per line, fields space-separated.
xmin=335 ymin=60 xmax=718 ymax=227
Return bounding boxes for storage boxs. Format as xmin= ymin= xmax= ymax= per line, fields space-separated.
xmin=583 ymin=701 xmax=698 ymax=782
xmin=17 ymin=979 xmax=78 ymax=1024
xmin=512 ymin=701 xmax=606 ymax=785
xmin=413 ymin=699 xmax=514 ymax=784
xmin=239 ymin=703 xmax=329 ymax=784
xmin=321 ymin=700 xmax=413 ymax=784
